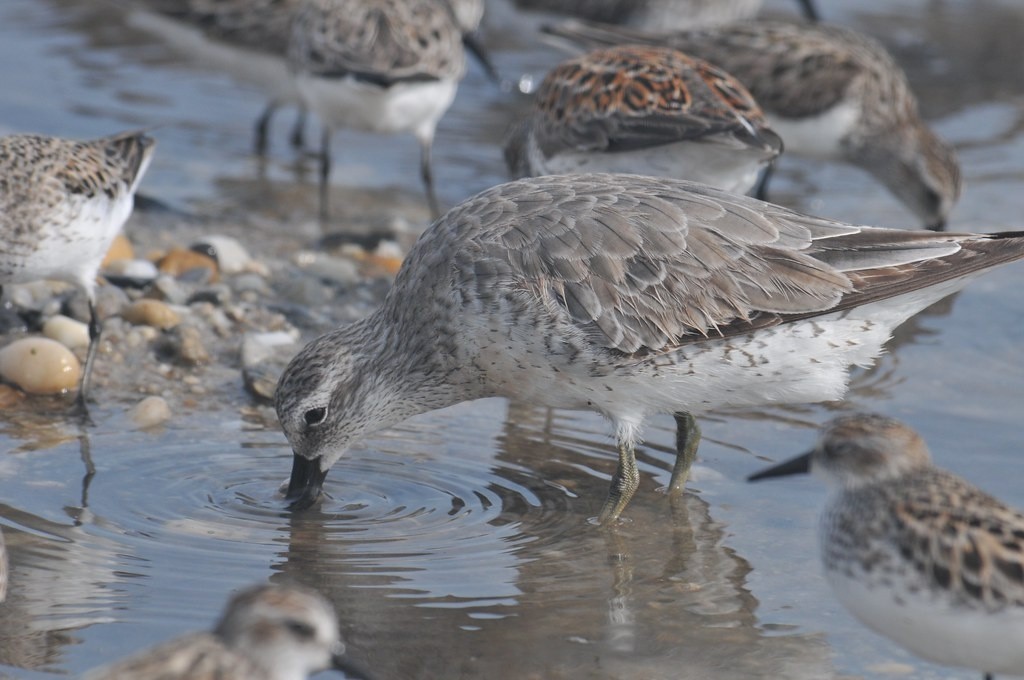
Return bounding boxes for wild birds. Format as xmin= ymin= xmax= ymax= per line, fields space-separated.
xmin=274 ymin=171 xmax=1024 ymax=523
xmin=0 ymin=126 xmax=158 ymax=426
xmin=115 ymin=2 xmax=501 ymax=178
xmin=506 ymin=3 xmax=965 ymax=232
xmin=75 ymin=582 xmax=369 ymax=679
xmin=746 ymin=413 xmax=1023 ymax=678
